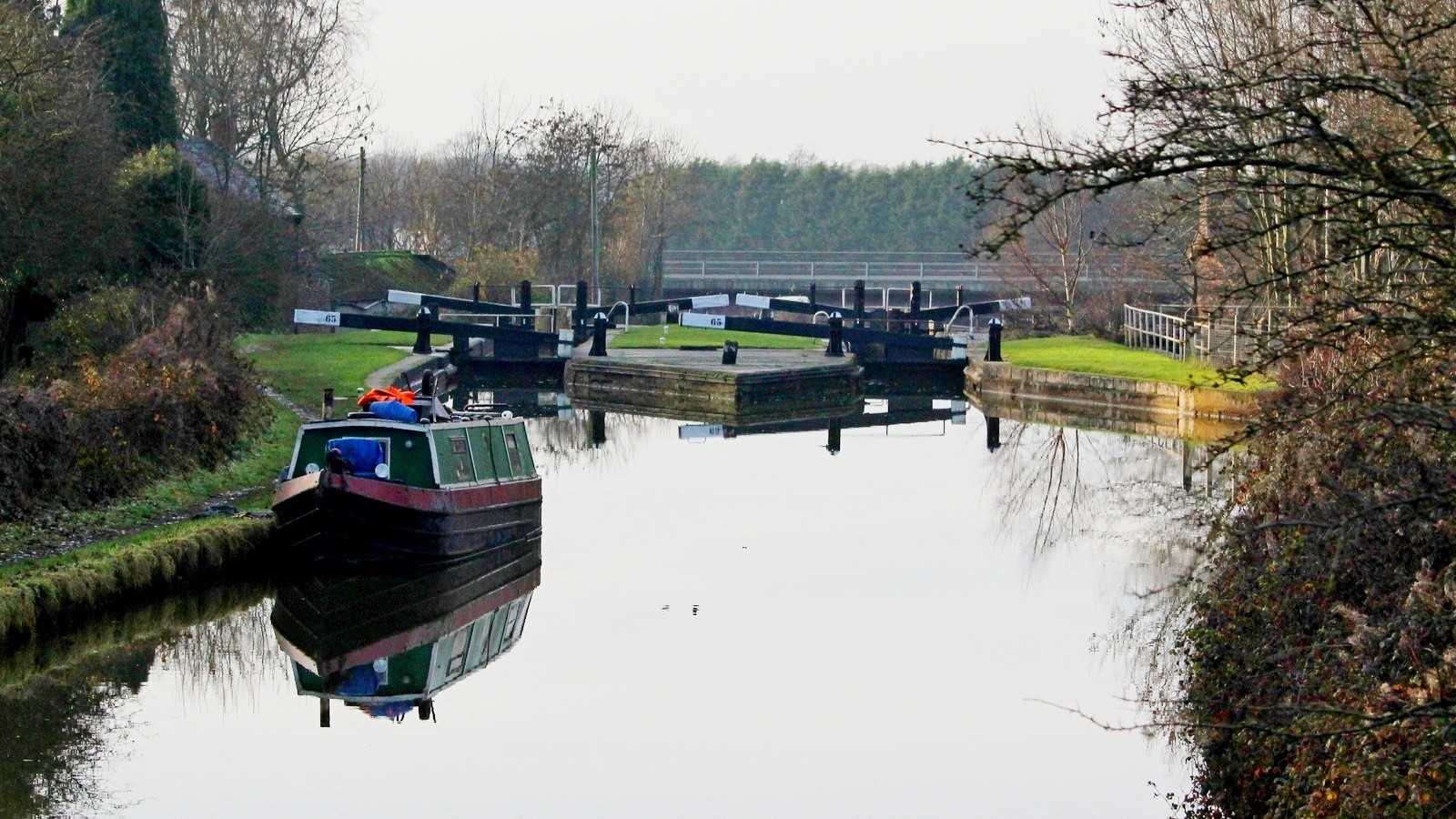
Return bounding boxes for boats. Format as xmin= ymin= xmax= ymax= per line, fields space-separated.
xmin=270 ymin=539 xmax=542 ymax=728
xmin=274 ymin=372 xmax=540 ymax=564
